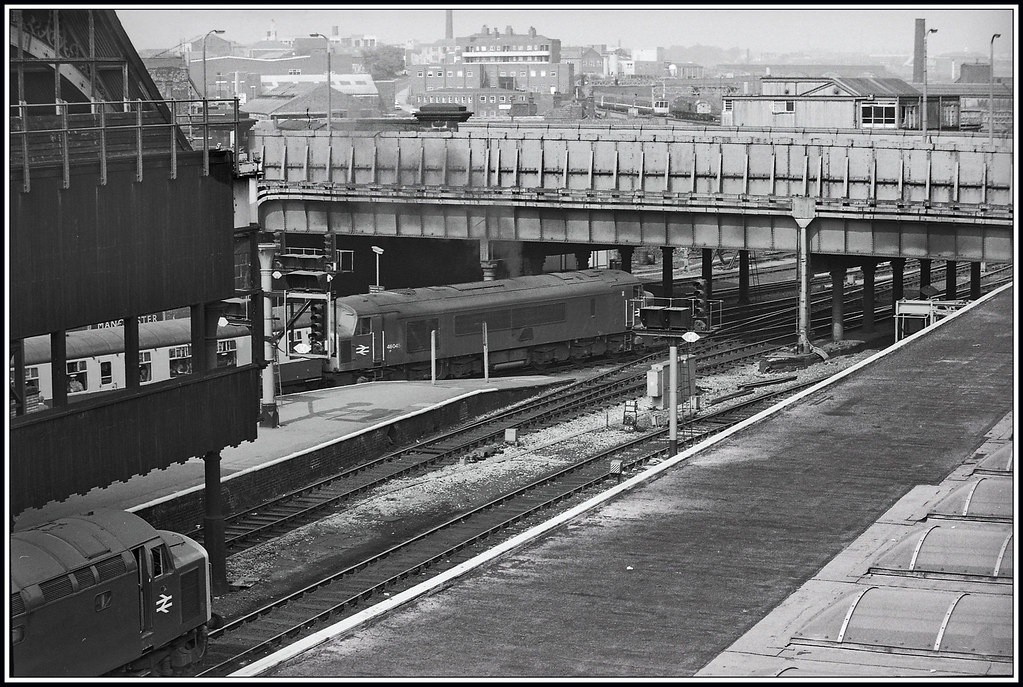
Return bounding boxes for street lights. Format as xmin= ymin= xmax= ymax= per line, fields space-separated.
xmin=204 ymin=30 xmax=226 ymax=122
xmin=989 ymin=33 xmax=1000 ymax=145
xmin=310 ymin=33 xmax=331 ymax=132
xmin=924 ymin=29 xmax=938 ymax=141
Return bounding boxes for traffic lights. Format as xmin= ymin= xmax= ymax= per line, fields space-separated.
xmin=694 ymin=278 xmax=707 ymax=317
xmin=323 ymin=231 xmax=337 ymax=263
xmin=308 ymin=299 xmax=328 ymax=341
xmin=273 ymin=229 xmax=285 ymax=253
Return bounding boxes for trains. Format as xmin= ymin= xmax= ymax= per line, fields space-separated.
xmin=10 ymin=266 xmax=654 ymax=425
xmin=9 ymin=511 xmax=211 ymax=678
